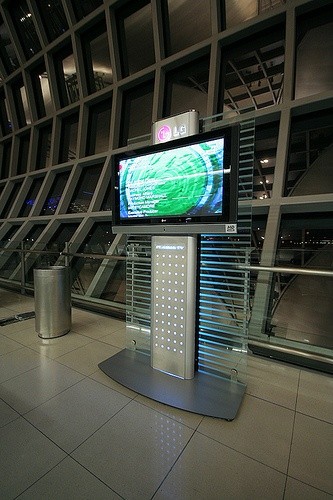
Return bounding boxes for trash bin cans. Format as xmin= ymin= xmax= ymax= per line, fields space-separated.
xmin=32 ymin=264 xmax=74 ymax=339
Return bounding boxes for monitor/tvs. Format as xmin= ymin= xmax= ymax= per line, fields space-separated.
xmin=110 ymin=122 xmax=239 ymax=237
